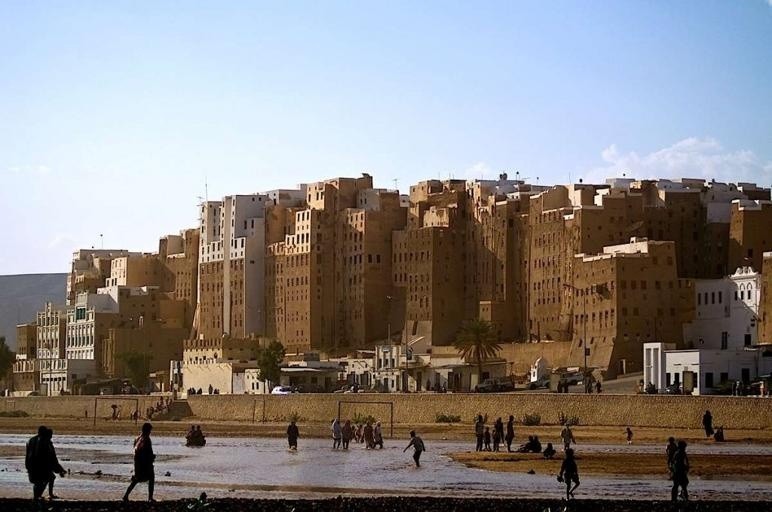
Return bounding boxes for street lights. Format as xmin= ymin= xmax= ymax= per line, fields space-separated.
xmin=562 ymin=284 xmax=588 ymax=394
xmin=386 ymin=294 xmax=409 ymax=393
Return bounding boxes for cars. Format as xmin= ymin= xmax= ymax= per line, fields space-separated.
xmin=270 ymin=386 xmax=299 ymax=394
xmin=333 ymin=385 xmax=369 ymax=393
xmin=541 ymin=370 xmax=583 ymax=388
xmin=714 ymin=373 xmax=771 ymax=395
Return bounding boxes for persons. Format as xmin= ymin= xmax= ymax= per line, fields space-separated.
xmin=25 ymin=426 xmax=67 ymax=503
xmin=287 ymin=421 xmax=300 ymax=450
xmin=331 ymin=419 xmax=384 ymax=450
xmin=403 ymin=430 xmax=425 ymax=467
xmin=703 ymin=410 xmax=714 ymax=437
xmin=714 ymin=429 xmax=722 ymax=442
xmin=122 ymin=422 xmax=157 ymax=502
xmin=111 ymin=381 xmax=219 ymax=438
xmin=557 ymin=448 xmax=580 ymax=499
xmin=736 ymin=377 xmax=742 ymax=396
xmin=556 ymin=379 xmax=601 ymax=393
xmin=665 ymin=437 xmax=689 ymax=502
xmin=427 ymin=377 xmax=446 ymax=393
xmin=474 ymin=414 xmax=576 ymax=458
xmin=623 ymin=428 xmax=633 ymax=444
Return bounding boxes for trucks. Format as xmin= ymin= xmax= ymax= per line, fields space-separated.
xmin=475 ymin=376 xmax=514 ymax=392
